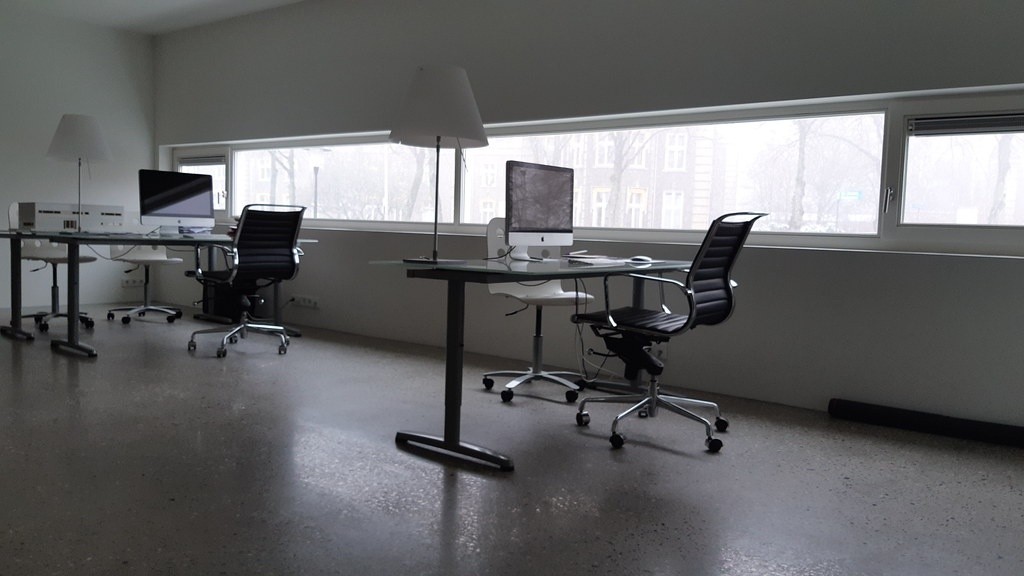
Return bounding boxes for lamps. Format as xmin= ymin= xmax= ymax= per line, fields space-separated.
xmin=388 ymin=63 xmax=489 ymax=266
xmin=45 ymin=113 xmax=114 ymax=235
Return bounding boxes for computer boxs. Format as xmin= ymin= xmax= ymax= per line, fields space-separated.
xmin=203 ymin=270 xmax=257 ymax=323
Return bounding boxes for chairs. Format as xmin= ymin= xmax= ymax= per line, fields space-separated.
xmin=8 ymin=202 xmax=95 ymax=333
xmin=106 ymin=211 xmax=183 ymax=325
xmin=570 ymin=211 xmax=768 ymax=458
xmin=482 ymin=217 xmax=586 ymax=403
xmin=184 ymin=203 xmax=307 ymax=359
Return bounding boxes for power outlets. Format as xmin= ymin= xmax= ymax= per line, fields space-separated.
xmin=643 ymin=342 xmax=669 ymax=360
xmin=121 ymin=278 xmax=145 ymax=288
xmin=290 ymin=293 xmax=320 ymax=308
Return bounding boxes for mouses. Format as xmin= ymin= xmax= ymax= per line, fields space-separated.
xmin=631 ymin=255 xmax=652 ymax=262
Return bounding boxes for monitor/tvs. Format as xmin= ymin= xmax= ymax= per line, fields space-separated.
xmin=139 ymin=168 xmax=216 ymax=236
xmin=506 ymin=160 xmax=574 ymax=261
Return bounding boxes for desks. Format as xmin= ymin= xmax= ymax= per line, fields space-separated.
xmin=31 ymin=232 xmax=318 ymax=361
xmin=0 ymin=230 xmax=235 ymax=343
xmin=395 ymin=259 xmax=688 ymax=474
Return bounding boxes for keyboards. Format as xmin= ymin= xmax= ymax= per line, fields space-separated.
xmin=183 ymin=234 xmax=232 ymax=239
xmin=569 ymin=258 xmax=625 ymax=266
